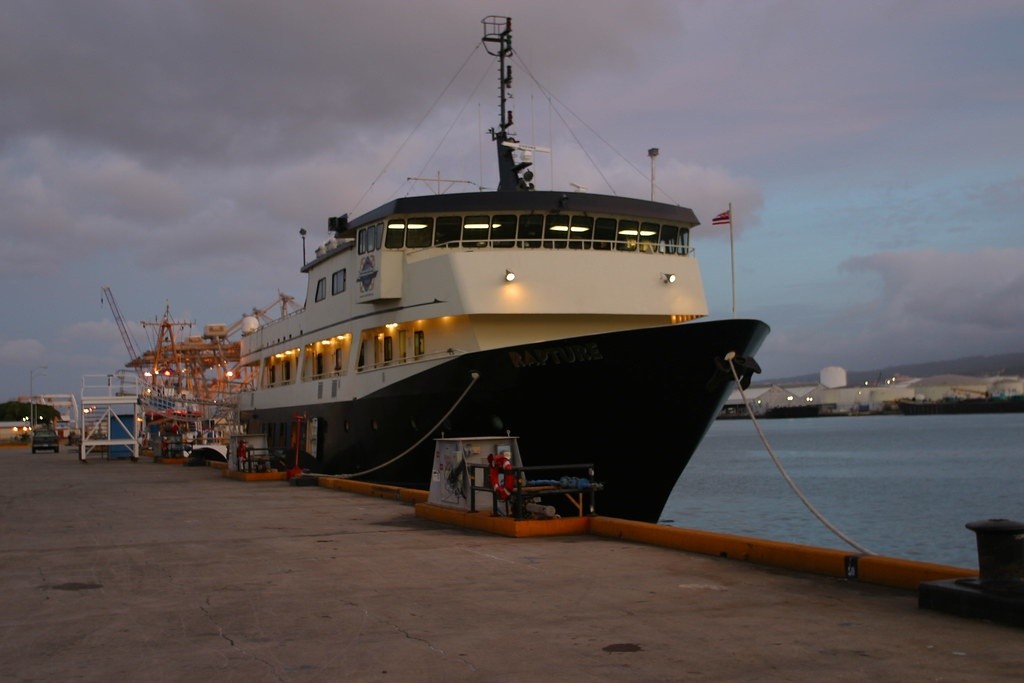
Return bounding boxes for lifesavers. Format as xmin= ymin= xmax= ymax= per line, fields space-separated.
xmin=489 ymin=455 xmax=514 ymax=499
xmin=238 ymin=443 xmax=246 ymax=462
xmin=162 ymin=436 xmax=167 ymax=451
xmin=141 ymin=432 xmax=147 ymax=447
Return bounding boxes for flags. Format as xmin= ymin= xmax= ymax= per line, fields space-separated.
xmin=711 ymin=210 xmax=731 ymax=225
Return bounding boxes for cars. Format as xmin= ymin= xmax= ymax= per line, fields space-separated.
xmin=32 ymin=429 xmax=59 ymax=453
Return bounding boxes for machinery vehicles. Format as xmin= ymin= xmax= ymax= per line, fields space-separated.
xmin=99 ymin=285 xmax=140 ymax=361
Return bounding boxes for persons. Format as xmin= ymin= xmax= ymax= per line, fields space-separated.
xmin=237 ymin=439 xmax=247 ymax=472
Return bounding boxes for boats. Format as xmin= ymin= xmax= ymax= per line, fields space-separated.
xmin=239 ymin=15 xmax=771 ymax=524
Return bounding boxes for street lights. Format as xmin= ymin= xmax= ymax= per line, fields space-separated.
xmin=30 ymin=366 xmax=49 ymax=428
